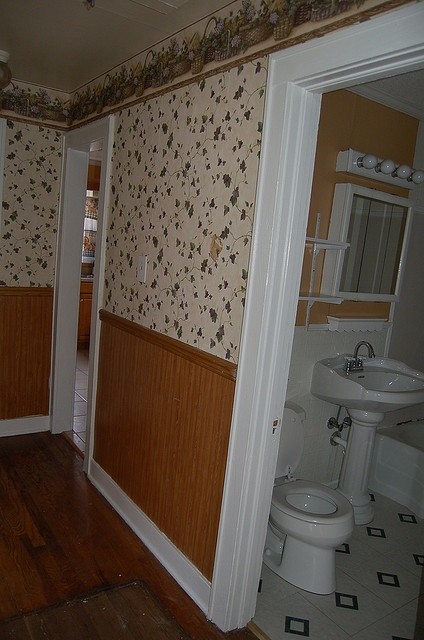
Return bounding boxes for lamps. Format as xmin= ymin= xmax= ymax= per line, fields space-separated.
xmin=407 ymin=170 xmax=424 ymax=184
xmin=375 ymin=158 xmax=395 ymax=174
xmin=357 ymin=153 xmax=378 ymax=169
xmin=391 ymin=164 xmax=411 ymax=179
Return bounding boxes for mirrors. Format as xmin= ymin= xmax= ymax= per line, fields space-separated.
xmin=320 ymin=182 xmax=415 ymax=302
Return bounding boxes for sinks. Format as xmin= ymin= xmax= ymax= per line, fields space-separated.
xmin=310 ymin=356 xmax=424 ymax=413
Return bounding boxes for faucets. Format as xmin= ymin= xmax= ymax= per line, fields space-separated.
xmin=354 ymin=341 xmax=375 ymax=367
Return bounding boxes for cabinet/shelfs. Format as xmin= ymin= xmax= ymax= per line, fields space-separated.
xmin=326 ymin=316 xmax=389 ymax=332
xmin=77 ymin=282 xmax=92 ymax=349
xmin=298 ymin=212 xmax=350 ymax=333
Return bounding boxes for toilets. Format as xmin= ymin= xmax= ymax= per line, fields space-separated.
xmin=263 ymin=401 xmax=354 ymax=595
